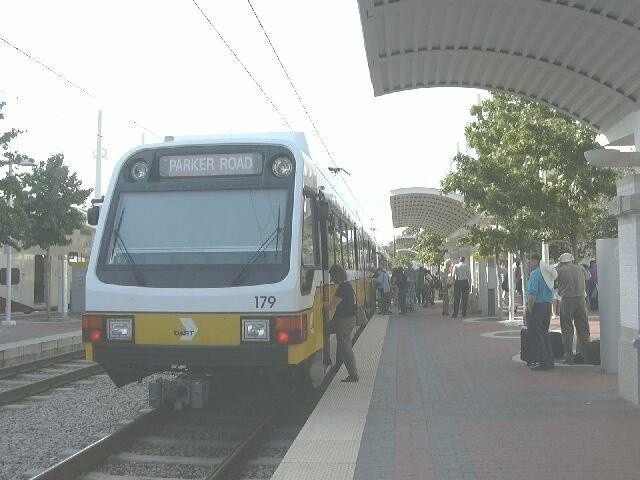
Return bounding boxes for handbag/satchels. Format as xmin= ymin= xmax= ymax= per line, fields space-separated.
xmin=324 ymin=315 xmax=338 ymax=335
xmin=354 ymin=294 xmax=368 ymax=327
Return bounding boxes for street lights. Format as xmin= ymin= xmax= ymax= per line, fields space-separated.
xmin=0 ymin=156 xmax=34 ymax=327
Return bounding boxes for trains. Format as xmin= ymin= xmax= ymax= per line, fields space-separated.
xmin=0 ymin=209 xmax=96 ymax=320
xmin=82 ymin=131 xmax=396 ymax=413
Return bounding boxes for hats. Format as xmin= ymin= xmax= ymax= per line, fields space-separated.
xmin=559 ymin=251 xmax=575 ymax=263
xmin=539 ymin=258 xmax=558 ymax=289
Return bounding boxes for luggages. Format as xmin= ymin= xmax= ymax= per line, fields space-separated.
xmin=586 ymin=339 xmax=600 ymax=366
xmin=549 ymin=330 xmax=562 ymax=363
xmin=521 ymin=326 xmax=537 ymax=364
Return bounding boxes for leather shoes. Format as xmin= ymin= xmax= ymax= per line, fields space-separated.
xmin=574 ymin=358 xmax=584 ymax=364
xmin=340 ymin=375 xmax=360 ymax=382
xmin=324 ymin=358 xmax=332 ymax=366
xmin=530 ymin=366 xmax=543 ymax=371
xmin=560 ymin=359 xmax=574 ymax=365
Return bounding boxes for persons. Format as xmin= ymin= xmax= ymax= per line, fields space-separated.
xmin=522 ymin=254 xmax=555 ymax=371
xmin=552 ymin=253 xmax=593 ymax=364
xmin=440 ymin=258 xmax=453 ymax=316
xmin=367 ymin=264 xmax=439 ymax=316
xmin=320 ymin=264 xmax=362 ymax=383
xmin=451 ymin=256 xmax=471 ymax=317
xmin=498 ymin=260 xmax=597 ymax=316
